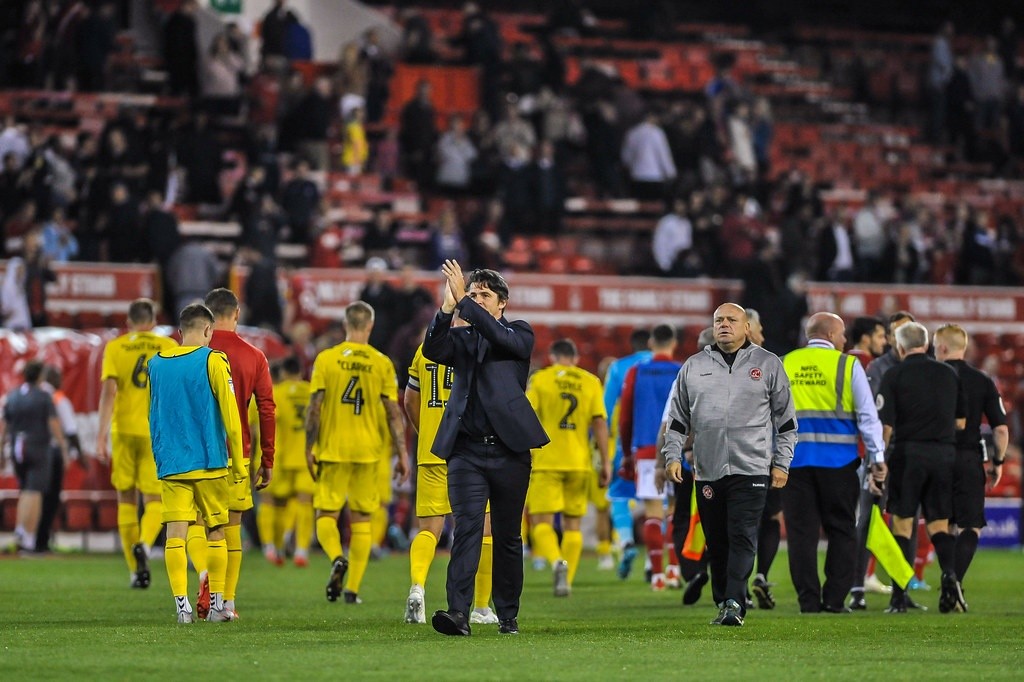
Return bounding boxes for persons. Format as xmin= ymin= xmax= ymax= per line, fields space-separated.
xmin=95 ymin=301 xmax=181 ymax=589
xmin=662 ymin=301 xmax=799 ymax=630
xmin=592 ymin=309 xmax=788 ymax=608
xmin=1 ymin=358 xmax=72 ymax=554
xmin=839 ymin=309 xmax=1009 ymax=614
xmin=243 ymin=352 xmax=394 ymax=567
xmin=138 ymin=303 xmax=249 ymax=625
xmin=773 ymin=311 xmax=888 ymax=615
xmin=170 ymin=288 xmax=277 ymax=619
xmin=522 ymin=337 xmax=613 ymax=600
xmin=399 ymin=313 xmax=502 ymax=628
xmin=0 ymin=0 xmax=1024 ymax=354
xmin=419 ymin=258 xmax=551 ymax=636
xmin=303 ymin=300 xmax=410 ymax=606
xmin=18 ymin=366 xmax=92 ymax=557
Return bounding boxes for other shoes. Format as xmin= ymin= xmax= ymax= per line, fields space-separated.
xmin=710 ymin=599 xmax=745 ymax=627
xmin=847 ymin=587 xmax=867 ymax=609
xmin=177 ymin=610 xmax=193 ymax=625
xmin=129 ymin=542 xmax=152 ymax=589
xmin=683 ymin=572 xmax=710 ymax=605
xmin=864 ymin=574 xmax=892 ymax=595
xmin=617 ymin=545 xmax=638 ymax=578
xmin=206 ymin=607 xmax=234 ymax=623
xmin=325 ymin=556 xmax=349 ymax=602
xmin=751 ymin=578 xmax=777 ymax=609
xmin=938 ymin=570 xmax=968 ymax=613
xmin=195 ymin=571 xmax=211 ymax=619
xmin=345 ymin=592 xmax=363 ymax=604
xmin=665 ymin=565 xmax=683 ymax=588
xmin=553 ymin=559 xmax=570 ymax=598
xmin=907 ymin=575 xmax=932 ymax=591
xmin=651 ymin=573 xmax=667 ymax=591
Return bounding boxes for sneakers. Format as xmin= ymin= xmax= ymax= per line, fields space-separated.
xmin=404 ymin=587 xmax=426 ymax=624
xmin=470 ymin=606 xmax=499 ymax=625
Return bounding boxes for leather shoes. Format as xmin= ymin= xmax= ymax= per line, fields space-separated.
xmin=497 ymin=618 xmax=519 ymax=634
xmin=432 ymin=609 xmax=472 ymax=636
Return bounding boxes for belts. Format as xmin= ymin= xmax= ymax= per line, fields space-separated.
xmin=458 ymin=435 xmax=500 ymax=446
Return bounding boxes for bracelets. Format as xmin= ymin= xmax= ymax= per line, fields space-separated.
xmin=992 ymin=455 xmax=1006 ymax=466
